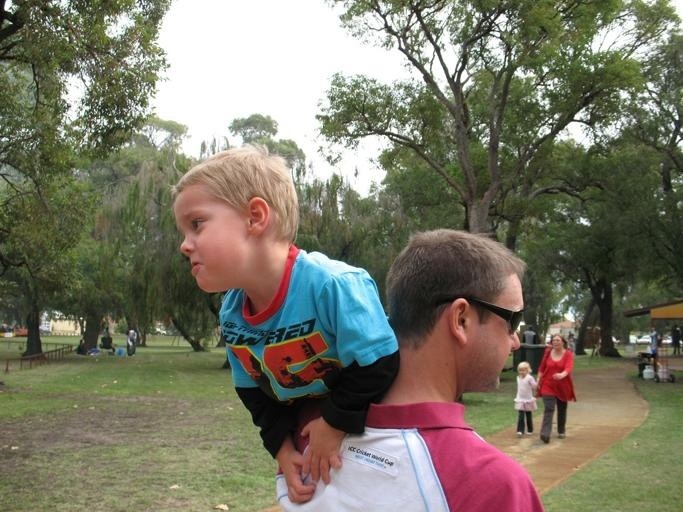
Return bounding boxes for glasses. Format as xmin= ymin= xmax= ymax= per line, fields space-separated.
xmin=433 ymin=293 xmax=525 ymax=332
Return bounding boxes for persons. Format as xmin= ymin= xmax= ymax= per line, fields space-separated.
xmin=511 ymin=359 xmax=538 ymax=437
xmin=99 ymin=332 xmax=116 ymax=356
xmin=670 ymin=324 xmax=682 ymax=355
xmin=276 ymin=225 xmax=547 ymax=512
xmin=522 ymin=326 xmax=537 ymax=344
xmin=566 ymin=333 xmax=575 ymax=353
xmin=535 ymin=332 xmax=578 ymax=445
xmin=648 ymin=326 xmax=658 ymax=350
xmin=127 ymin=328 xmax=137 ymax=359
xmin=76 ymin=339 xmax=88 ymax=356
xmin=171 ymin=139 xmax=401 ymax=505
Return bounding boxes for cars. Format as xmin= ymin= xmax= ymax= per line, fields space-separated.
xmin=153 ymin=322 xmax=166 ymax=336
xmin=0 ymin=322 xmax=51 ymax=338
xmin=611 ymin=333 xmax=683 ymax=345
xmin=544 ymin=333 xmax=552 ymax=345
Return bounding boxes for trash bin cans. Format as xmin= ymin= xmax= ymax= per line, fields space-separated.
xmin=513 ymin=343 xmax=546 ymax=374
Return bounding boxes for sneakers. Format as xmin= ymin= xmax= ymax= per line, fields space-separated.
xmin=515 ymin=431 xmax=532 ymax=437
xmin=539 ymin=431 xmax=565 ymax=443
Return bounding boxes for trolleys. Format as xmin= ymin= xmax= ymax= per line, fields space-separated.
xmin=652 ymin=332 xmax=675 ymax=384
